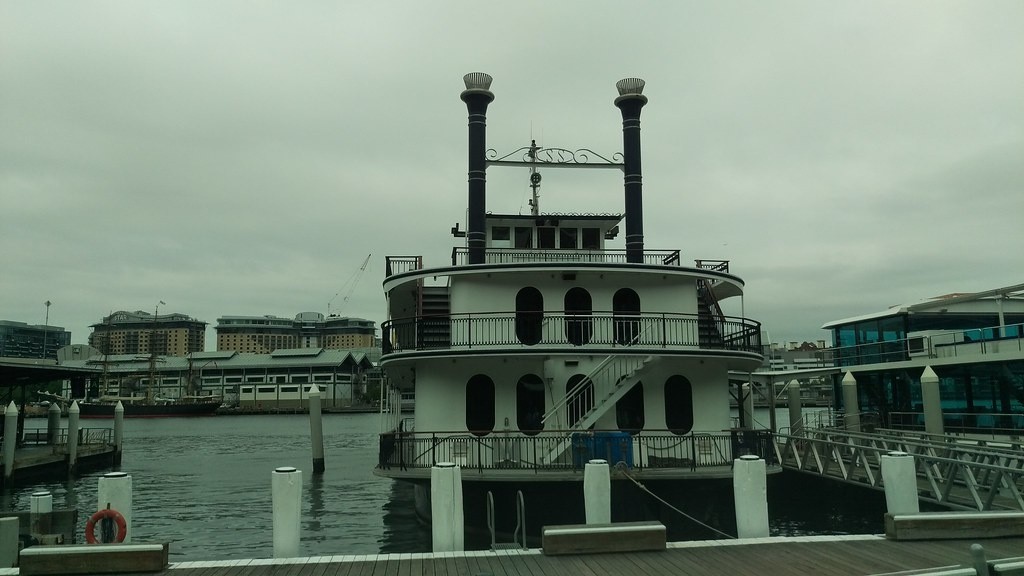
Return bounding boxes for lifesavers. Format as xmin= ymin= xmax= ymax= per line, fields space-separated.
xmin=85 ymin=509 xmax=127 ymax=545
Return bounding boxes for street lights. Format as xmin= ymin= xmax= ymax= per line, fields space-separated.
xmin=148 ymin=299 xmax=168 ymax=399
xmin=40 ymin=299 xmax=54 ymax=359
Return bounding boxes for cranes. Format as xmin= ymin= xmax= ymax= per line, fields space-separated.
xmin=324 ymin=254 xmax=371 ymax=318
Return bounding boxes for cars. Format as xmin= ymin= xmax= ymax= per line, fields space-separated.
xmin=381 ymin=393 xmax=415 ymax=410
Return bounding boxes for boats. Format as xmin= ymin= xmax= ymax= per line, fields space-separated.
xmin=65 ymin=395 xmax=224 ymax=419
xmin=373 ymin=71 xmax=781 ymax=529
xmin=823 ymin=286 xmax=1024 ymax=451
